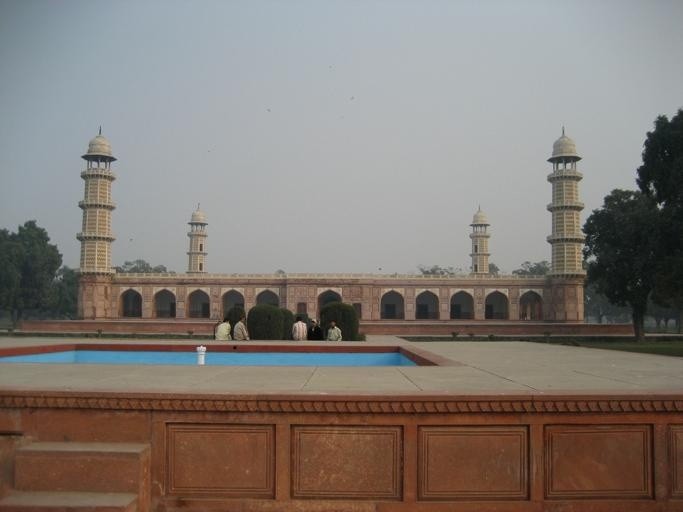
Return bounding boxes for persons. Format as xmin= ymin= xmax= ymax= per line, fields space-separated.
xmin=233 ymin=316 xmax=250 ymax=340
xmin=326 ymin=320 xmax=342 ymax=341
xmin=292 ymin=316 xmax=323 ymax=340
xmin=215 ymin=317 xmax=232 ymax=341
